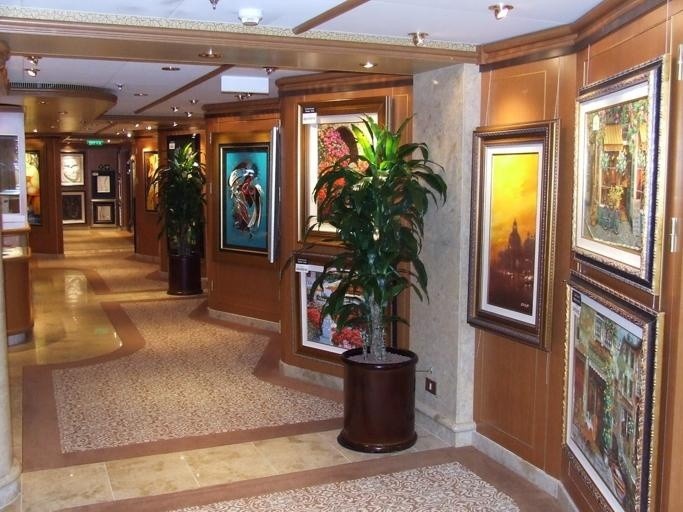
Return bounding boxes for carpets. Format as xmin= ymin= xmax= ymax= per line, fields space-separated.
xmin=21 ymin=295 xmax=344 ymax=472
xmin=37 ymin=252 xmax=207 ymax=295
xmin=51 ymin=446 xmax=570 ymax=512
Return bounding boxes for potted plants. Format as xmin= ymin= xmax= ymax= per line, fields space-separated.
xmin=281 ymin=112 xmax=447 ymax=453
xmin=151 ymin=142 xmax=207 ymax=295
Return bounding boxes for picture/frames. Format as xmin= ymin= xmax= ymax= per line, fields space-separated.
xmin=291 ymin=252 xmax=396 ymax=368
xmin=60 ymin=150 xmax=117 ymax=228
xmin=143 ymin=151 xmax=159 ymax=212
xmin=572 ymin=54 xmax=672 ymax=296
xmin=295 ymin=94 xmax=392 ymax=247
xmin=562 ymin=268 xmax=666 ymax=512
xmin=26 ymin=142 xmax=50 ymax=233
xmin=270 ymin=127 xmax=281 ymax=264
xmin=467 ymin=119 xmax=561 ymax=352
xmin=219 ymin=142 xmax=270 ymax=257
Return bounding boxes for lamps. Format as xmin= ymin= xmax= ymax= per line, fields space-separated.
xmin=26 ymin=57 xmax=275 ymax=119
xmin=408 ymin=32 xmax=430 ymax=48
xmin=489 ymin=5 xmax=514 ymax=19
